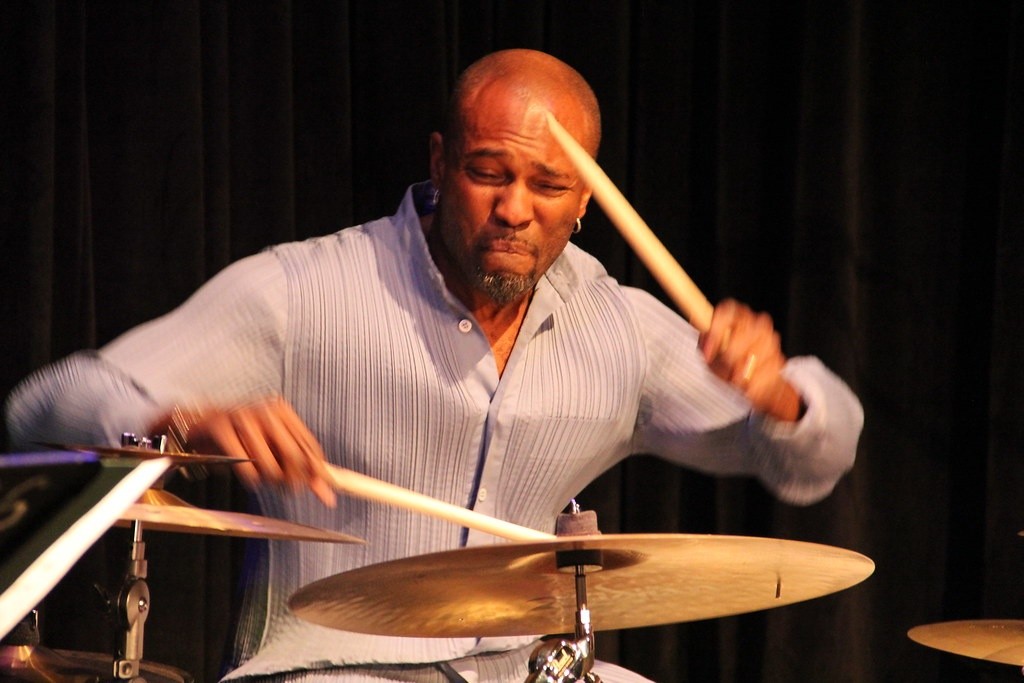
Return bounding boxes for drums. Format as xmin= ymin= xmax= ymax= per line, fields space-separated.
xmin=0 ymin=644 xmax=199 ymax=683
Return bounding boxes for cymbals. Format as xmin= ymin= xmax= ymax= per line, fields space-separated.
xmin=23 ymin=435 xmax=252 ymax=465
xmin=282 ymin=532 xmax=878 ymax=641
xmin=15 ymin=480 xmax=371 ymax=547
xmin=905 ymin=617 xmax=1023 ymax=668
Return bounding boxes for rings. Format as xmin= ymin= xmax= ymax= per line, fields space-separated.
xmin=743 ymin=352 xmax=759 ymax=365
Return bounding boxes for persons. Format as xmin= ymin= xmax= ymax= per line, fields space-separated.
xmin=11 ymin=48 xmax=866 ymax=683
